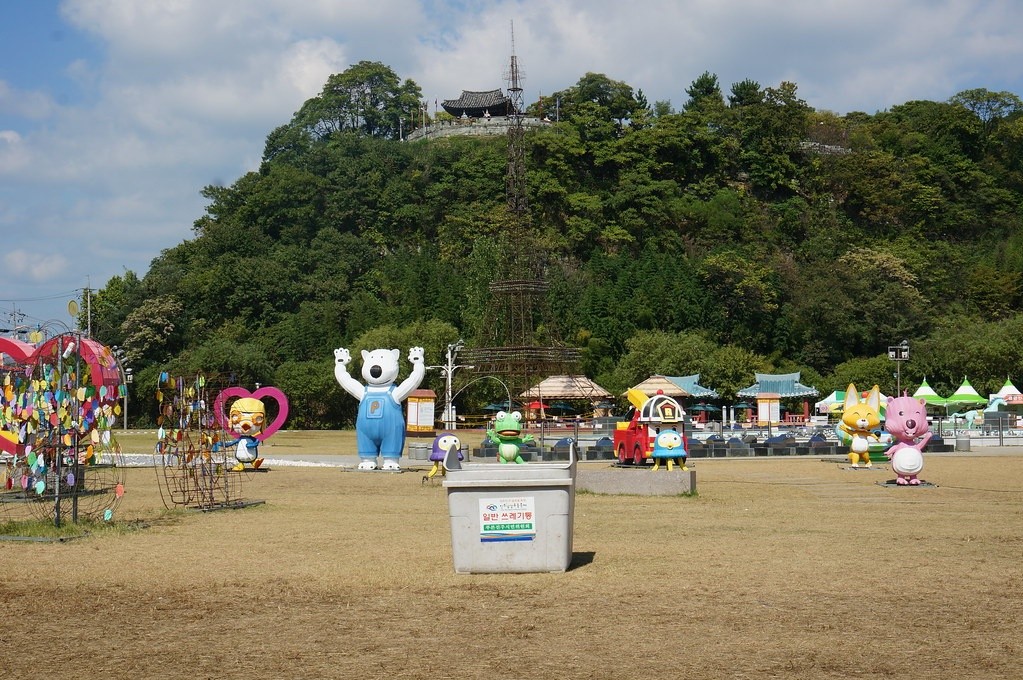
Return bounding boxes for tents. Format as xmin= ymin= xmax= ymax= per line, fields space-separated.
xmin=814 ymin=375 xmax=1023 ymax=420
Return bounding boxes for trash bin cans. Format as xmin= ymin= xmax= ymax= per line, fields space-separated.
xmin=442 ymin=442 xmax=578 ymax=574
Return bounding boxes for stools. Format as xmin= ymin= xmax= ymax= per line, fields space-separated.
xmin=956 ymin=434 xmax=970 ymax=451
xmin=409 ymin=442 xmax=469 ymax=463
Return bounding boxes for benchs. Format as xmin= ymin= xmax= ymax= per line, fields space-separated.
xmin=473 ymin=437 xmax=944 ymax=467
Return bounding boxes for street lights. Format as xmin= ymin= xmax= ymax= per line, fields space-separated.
xmin=897 ymin=339 xmax=908 ymax=398
xmin=425 ymin=339 xmax=475 ymax=430
